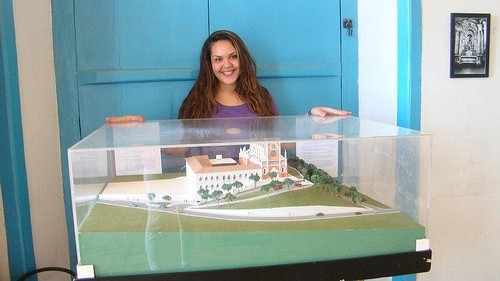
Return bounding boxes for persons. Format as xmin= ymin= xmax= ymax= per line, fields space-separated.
xmin=105 ymin=29 xmax=352 ymax=160
xmin=129 ymin=132 xmax=343 ymax=146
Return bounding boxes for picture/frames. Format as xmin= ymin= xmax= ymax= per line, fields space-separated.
xmin=449 ymin=12 xmax=490 ymax=77
